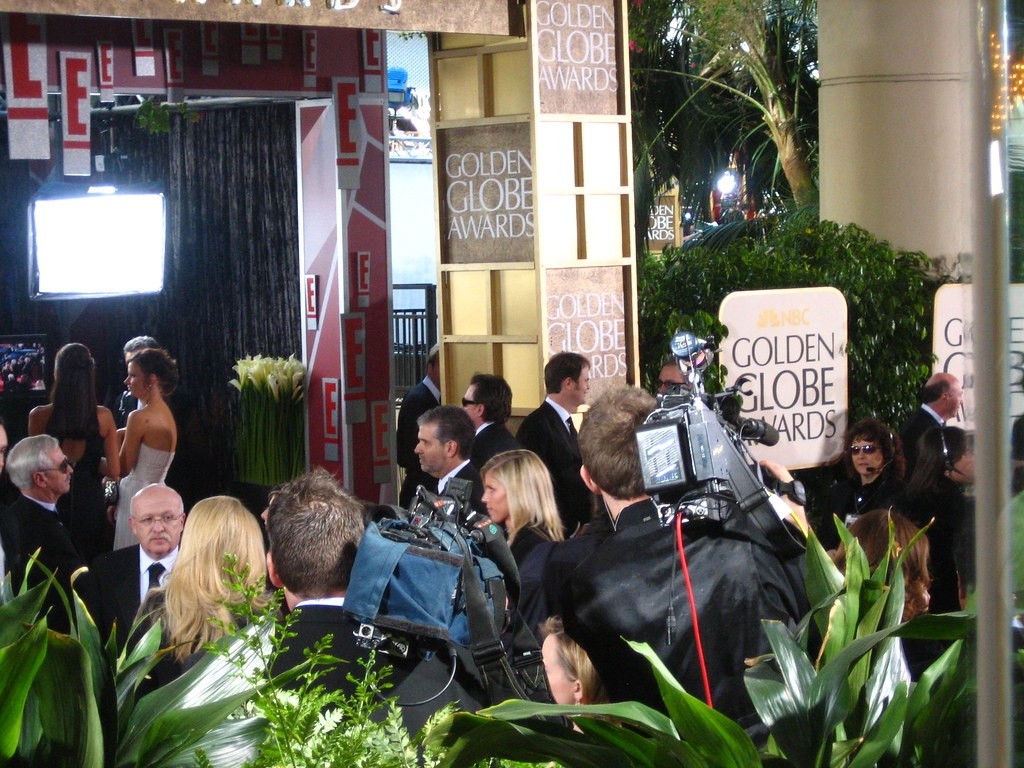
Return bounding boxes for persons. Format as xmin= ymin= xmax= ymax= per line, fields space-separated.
xmin=102 ymin=347 xmax=179 ymax=551
xmin=480 ymin=448 xmax=566 ymax=702
xmin=414 ymin=405 xmax=488 ymax=516
xmin=396 ymin=344 xmax=442 ymax=511
xmin=655 ymin=354 xmax=686 ymax=397
xmin=112 ymin=335 xmax=164 ymax=428
xmin=540 ymin=615 xmax=623 ymax=734
xmin=897 ymin=372 xmax=1024 ymax=656
xmin=115 ymin=495 xmax=283 ymax=708
xmin=0 ymin=434 xmax=85 ymax=634
xmin=462 ymin=373 xmax=513 ymax=471
xmin=86 ymin=483 xmax=186 ymax=659
xmin=184 ymin=460 xmax=496 ymax=741
xmin=562 ymin=383 xmax=808 ymax=731
xmin=514 ymin=350 xmax=593 ymax=542
xmin=817 ymin=417 xmax=906 ymax=561
xmin=0 ymin=419 xmax=8 ymax=475
xmin=28 ymin=342 xmax=120 ymax=640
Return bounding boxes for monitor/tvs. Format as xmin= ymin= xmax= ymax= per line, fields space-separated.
xmin=0 ymin=334 xmax=48 ymax=401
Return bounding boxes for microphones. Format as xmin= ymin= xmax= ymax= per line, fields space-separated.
xmin=866 ymin=459 xmax=895 ymax=473
xmin=744 ymin=418 xmax=780 ymax=446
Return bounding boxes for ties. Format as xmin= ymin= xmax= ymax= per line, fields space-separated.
xmin=567 ymin=417 xmax=579 ymax=454
xmin=145 ymin=563 xmax=166 ymax=591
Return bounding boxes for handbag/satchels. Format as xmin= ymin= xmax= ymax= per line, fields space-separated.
xmin=104 ymin=480 xmax=118 ymax=505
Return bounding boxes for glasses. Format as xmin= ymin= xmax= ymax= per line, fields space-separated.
xmin=655 ymin=379 xmax=687 ymax=389
xmin=851 ymin=445 xmax=884 ymax=455
xmin=135 ymin=513 xmax=183 ymax=525
xmin=462 ymin=397 xmax=475 ymax=406
xmin=37 ymin=457 xmax=69 ymax=473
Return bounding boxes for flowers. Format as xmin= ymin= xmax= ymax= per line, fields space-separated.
xmin=227 ymin=351 xmax=306 ymax=486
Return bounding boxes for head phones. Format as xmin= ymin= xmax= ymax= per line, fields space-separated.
xmin=883 ymin=430 xmax=896 ymax=463
xmin=939 ymin=428 xmax=954 ymax=472
